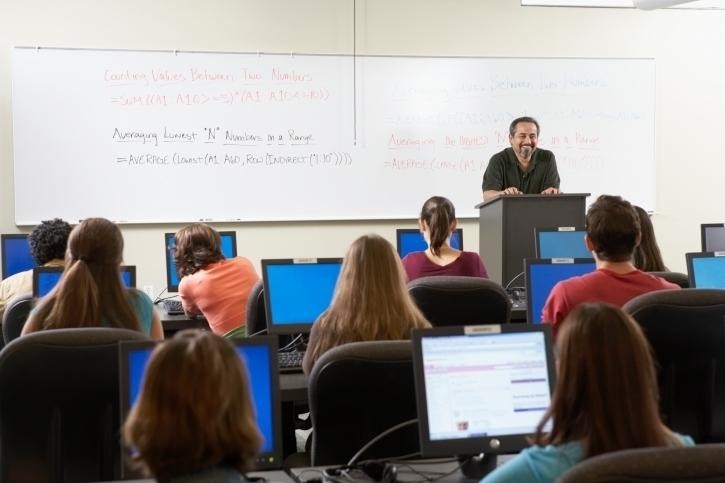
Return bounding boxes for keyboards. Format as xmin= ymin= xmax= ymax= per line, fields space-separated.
xmin=162 ymin=300 xmax=185 ymax=315
xmin=278 ymin=351 xmax=306 ymax=372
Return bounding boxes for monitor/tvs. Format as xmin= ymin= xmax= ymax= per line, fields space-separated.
xmin=118 ymin=334 xmax=284 ymax=480
xmin=396 ymin=229 xmax=463 ymax=258
xmin=164 ymin=231 xmax=237 ymax=292
xmin=410 ymin=324 xmax=560 ymax=478
xmin=523 ymin=223 xmax=725 ymax=324
xmin=33 ymin=266 xmax=136 ymax=300
xmin=261 ymin=258 xmax=351 ymax=335
xmin=0 ymin=233 xmax=39 ymax=280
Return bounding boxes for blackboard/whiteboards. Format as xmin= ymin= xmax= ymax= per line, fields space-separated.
xmin=12 ymin=45 xmax=660 ymax=226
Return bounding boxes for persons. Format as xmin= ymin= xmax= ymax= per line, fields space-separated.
xmin=399 ymin=193 xmax=487 ymax=285
xmin=171 ymin=223 xmax=263 ymax=340
xmin=15 ymin=219 xmax=165 ymax=342
xmin=476 ymin=293 xmax=695 ymax=482
xmin=0 ymin=216 xmax=70 ymax=319
xmin=482 ymin=115 xmax=562 ymax=199
xmin=300 ymin=235 xmax=435 ymax=373
xmin=627 ymin=201 xmax=666 ymax=274
xmin=117 ymin=327 xmax=265 ymax=483
xmin=541 ymin=194 xmax=683 ymax=328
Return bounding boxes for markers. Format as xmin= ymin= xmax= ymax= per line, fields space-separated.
xmin=199 ymin=219 xmax=212 ymax=221
